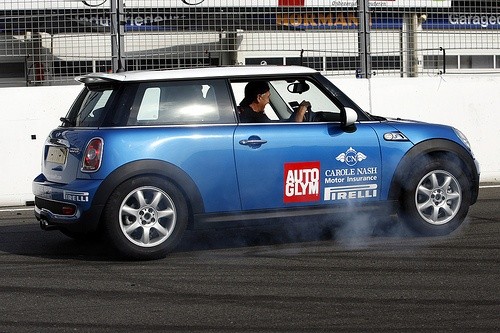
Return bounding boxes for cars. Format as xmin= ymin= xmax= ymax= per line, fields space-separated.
xmin=32 ymin=71 xmax=481 ymax=260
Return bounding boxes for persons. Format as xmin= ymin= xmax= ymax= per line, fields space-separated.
xmin=238 ymin=80 xmax=308 ymax=123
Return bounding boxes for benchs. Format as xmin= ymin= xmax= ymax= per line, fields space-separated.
xmin=37 ymin=32 xmax=500 ymax=68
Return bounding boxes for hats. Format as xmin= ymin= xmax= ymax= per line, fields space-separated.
xmin=239 ymin=80 xmax=269 ymax=107
